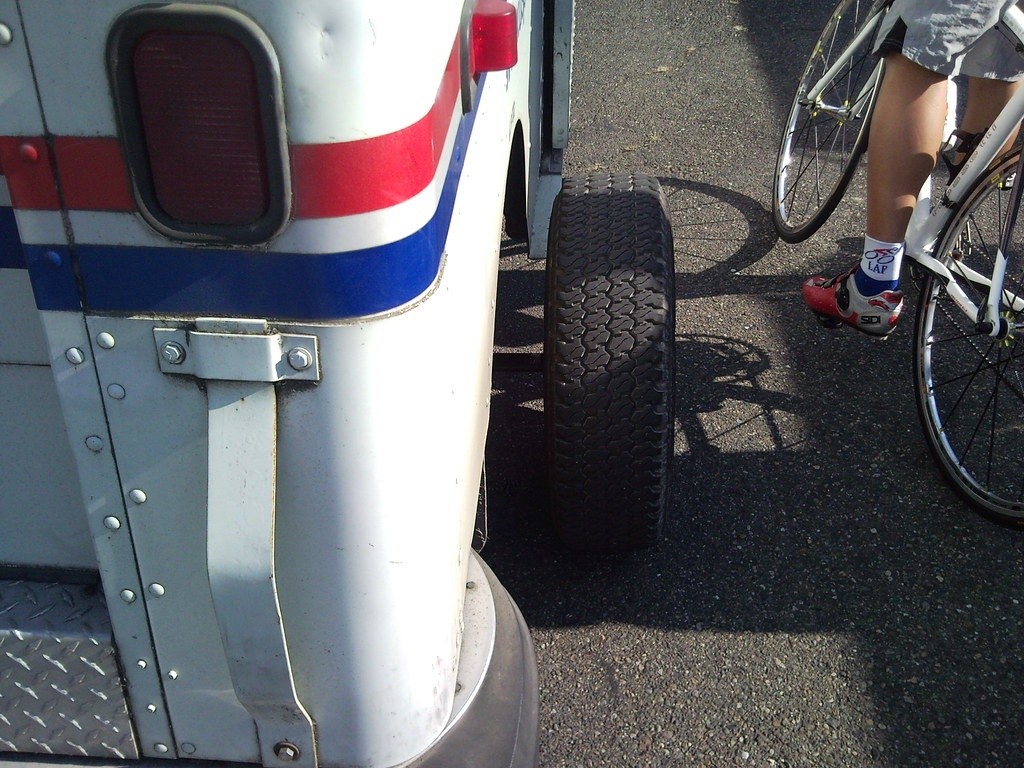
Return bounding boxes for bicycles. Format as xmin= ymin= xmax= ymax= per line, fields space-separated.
xmin=769 ymin=1 xmax=1024 ymax=530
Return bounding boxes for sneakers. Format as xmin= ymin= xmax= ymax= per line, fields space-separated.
xmin=802 ymin=265 xmax=905 ymax=340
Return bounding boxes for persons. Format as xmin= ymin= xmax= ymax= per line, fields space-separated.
xmin=800 ymin=0 xmax=1024 ymax=337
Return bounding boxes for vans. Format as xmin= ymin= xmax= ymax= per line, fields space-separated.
xmin=0 ymin=0 xmax=675 ymax=768
